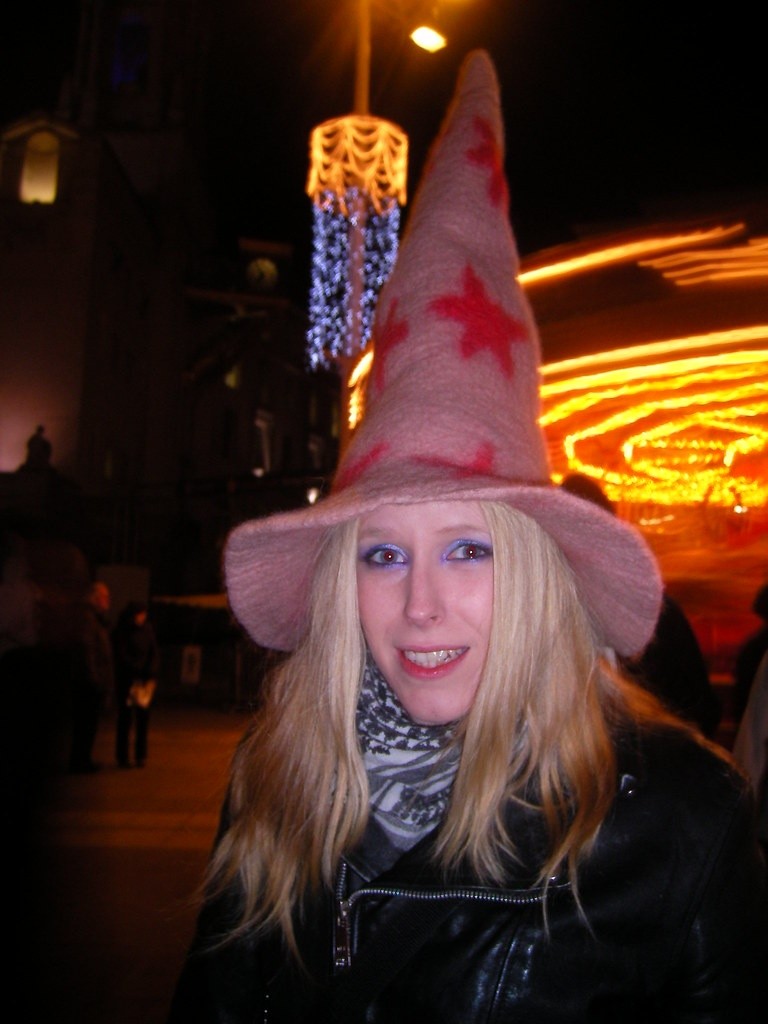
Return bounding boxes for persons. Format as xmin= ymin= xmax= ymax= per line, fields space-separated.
xmin=559 ymin=474 xmax=767 ymax=773
xmin=170 ymin=500 xmax=768 ymax=1024
xmin=27 ymin=426 xmax=51 ymax=466
xmin=0 ymin=506 xmax=171 ymax=773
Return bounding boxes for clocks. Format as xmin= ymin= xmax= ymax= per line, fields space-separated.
xmin=247 ymin=257 xmax=279 ymax=293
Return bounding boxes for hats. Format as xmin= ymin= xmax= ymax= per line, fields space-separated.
xmin=223 ymin=48 xmax=664 ymax=657
